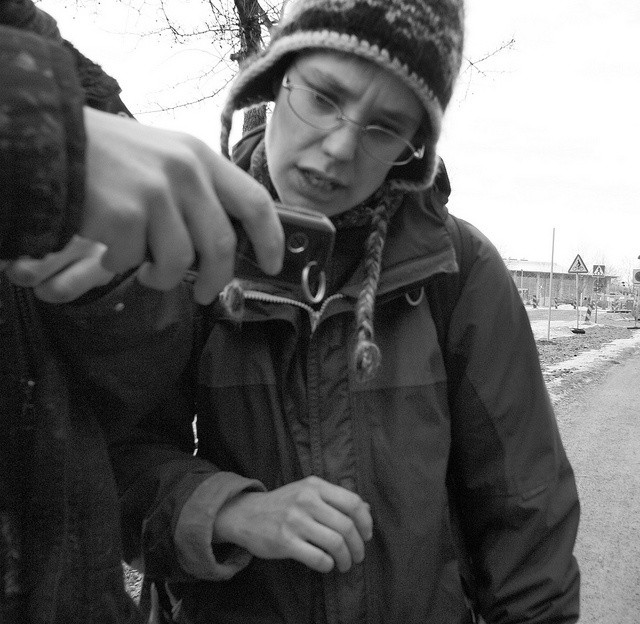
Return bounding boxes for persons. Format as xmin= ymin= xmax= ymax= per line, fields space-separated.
xmin=75 ymin=0 xmax=580 ymax=623
xmin=0 ymin=0 xmax=286 ymax=623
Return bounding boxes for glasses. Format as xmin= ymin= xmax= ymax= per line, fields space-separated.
xmin=283 ymin=73 xmax=425 ymax=166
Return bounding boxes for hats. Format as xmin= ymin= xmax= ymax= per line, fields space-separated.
xmin=220 ymin=0 xmax=466 ymax=380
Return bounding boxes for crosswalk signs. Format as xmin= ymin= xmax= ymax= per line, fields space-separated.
xmin=592 ymin=265 xmax=605 ymax=276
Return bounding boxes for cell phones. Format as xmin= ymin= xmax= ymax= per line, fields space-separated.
xmin=141 ymin=199 xmax=337 ymax=304
